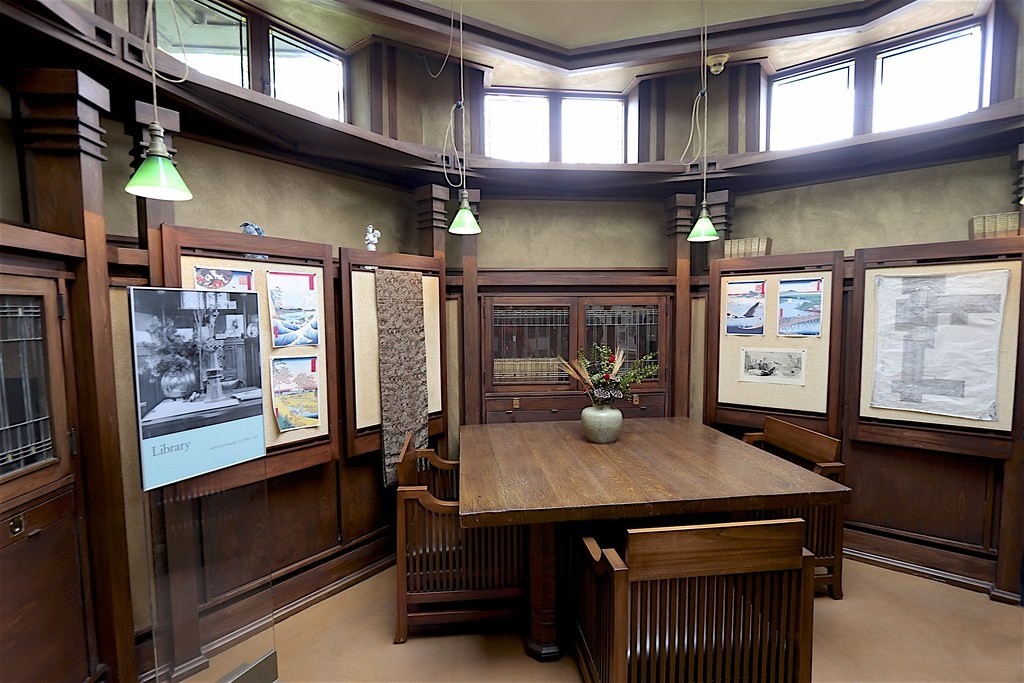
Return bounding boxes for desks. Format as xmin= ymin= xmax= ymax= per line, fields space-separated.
xmin=457 ymin=413 xmax=853 ymax=665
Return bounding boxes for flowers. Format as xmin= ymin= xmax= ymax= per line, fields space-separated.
xmin=554 ymin=338 xmax=661 ymax=406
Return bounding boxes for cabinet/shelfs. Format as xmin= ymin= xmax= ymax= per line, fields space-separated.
xmin=475 ymin=268 xmax=680 ymax=424
xmin=0 ymin=221 xmax=112 ymax=683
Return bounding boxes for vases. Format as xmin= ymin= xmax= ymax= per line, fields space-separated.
xmin=579 ymin=402 xmax=624 ymax=445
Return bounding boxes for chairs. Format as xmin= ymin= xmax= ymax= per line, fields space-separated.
xmin=565 ymin=514 xmax=817 ymax=683
xmin=738 ymin=414 xmax=848 ymax=600
xmin=390 ymin=428 xmax=527 ymax=645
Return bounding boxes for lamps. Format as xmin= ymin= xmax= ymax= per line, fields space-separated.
xmin=446 ymin=0 xmax=482 ymax=235
xmin=681 ymin=0 xmax=721 ymax=242
xmin=125 ymin=1 xmax=193 ymax=203
